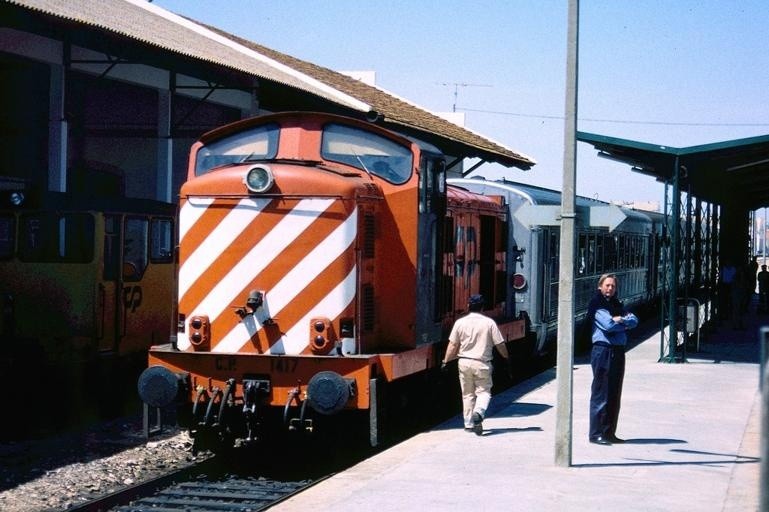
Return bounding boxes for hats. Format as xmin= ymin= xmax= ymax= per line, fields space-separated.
xmin=467 ymin=293 xmax=484 ymax=306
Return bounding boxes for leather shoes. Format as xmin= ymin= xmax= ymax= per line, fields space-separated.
xmin=607 ymin=435 xmax=624 ymax=443
xmin=590 ymin=436 xmax=610 ymax=445
xmin=473 ymin=413 xmax=483 ymax=435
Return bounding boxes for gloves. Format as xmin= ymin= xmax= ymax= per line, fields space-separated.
xmin=441 ymin=362 xmax=449 ymax=372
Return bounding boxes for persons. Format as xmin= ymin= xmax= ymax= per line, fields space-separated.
xmin=718 ymin=257 xmax=737 ymax=321
xmin=757 ymin=265 xmax=768 ymax=310
xmin=441 ymin=292 xmax=510 ymax=434
xmin=586 ymin=272 xmax=639 ymax=447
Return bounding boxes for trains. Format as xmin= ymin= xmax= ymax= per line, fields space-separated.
xmin=136 ymin=110 xmax=720 ymax=456
xmin=0 ymin=189 xmax=179 ymax=378
